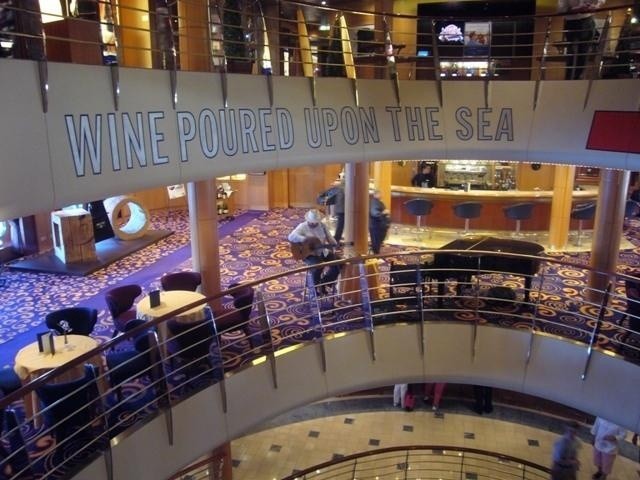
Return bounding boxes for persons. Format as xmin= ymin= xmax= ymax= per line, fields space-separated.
xmin=589 ymin=416 xmax=628 ymax=480
xmin=392 ymin=384 xmax=408 ymax=409
xmin=328 ymin=179 xmax=345 ymax=242
xmin=423 ymin=383 xmax=446 ymax=410
xmin=287 ymin=208 xmax=341 ymax=297
xmin=471 ymin=385 xmax=493 ymax=414
xmin=100 ymin=19 xmax=119 ymax=65
xmin=369 ymin=194 xmax=392 ymax=254
xmin=631 ymin=433 xmax=640 ymax=476
xmin=557 ymin=0 xmax=607 ymax=79
xmin=551 ymin=421 xmax=584 ymax=480
xmin=467 ymin=31 xmax=482 ymax=46
xmin=411 ymin=164 xmax=434 ymax=187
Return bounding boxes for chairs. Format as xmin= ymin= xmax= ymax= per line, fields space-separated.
xmin=107 ymin=270 xmax=256 ymax=379
xmin=45 ymin=306 xmax=98 ymax=336
xmin=105 ymin=320 xmax=161 ymax=412
xmin=1 ymin=368 xmax=22 ymax=398
xmin=403 ymin=198 xmax=535 ymax=238
xmin=624 ymin=200 xmax=640 ymax=237
xmin=571 ymin=200 xmax=595 ymax=247
xmin=35 ymin=367 xmax=100 ymax=459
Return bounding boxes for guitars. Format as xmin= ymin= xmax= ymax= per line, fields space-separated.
xmin=291 ymin=237 xmax=354 ymax=260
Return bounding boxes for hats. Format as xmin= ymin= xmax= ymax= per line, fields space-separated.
xmin=304 ymin=208 xmax=322 ymax=223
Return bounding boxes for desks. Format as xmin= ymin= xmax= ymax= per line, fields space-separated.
xmin=13 ymin=335 xmax=107 ymax=429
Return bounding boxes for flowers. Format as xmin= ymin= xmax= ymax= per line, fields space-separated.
xmin=57 ymin=320 xmax=73 ymax=351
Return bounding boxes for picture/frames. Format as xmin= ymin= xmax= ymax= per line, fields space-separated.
xmin=463 ymin=21 xmax=492 ymax=59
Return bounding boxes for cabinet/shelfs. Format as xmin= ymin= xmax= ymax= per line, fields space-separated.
xmin=217 ymin=188 xmax=239 ymax=221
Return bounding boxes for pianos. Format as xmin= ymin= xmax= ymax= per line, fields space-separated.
xmin=419 ymin=240 xmax=545 ymax=301
